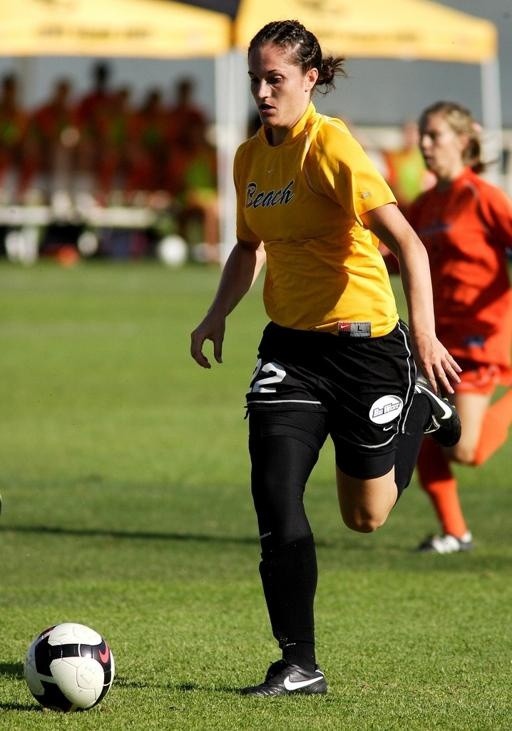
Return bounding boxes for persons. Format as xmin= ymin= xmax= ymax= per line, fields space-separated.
xmin=189 ymin=19 xmax=464 ymax=698
xmin=373 ymin=121 xmax=439 ymax=279
xmin=382 ymin=101 xmax=511 ymax=555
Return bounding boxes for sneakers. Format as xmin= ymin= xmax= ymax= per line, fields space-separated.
xmin=413 ymin=372 xmax=463 ymax=451
xmin=239 ymin=659 xmax=330 ymax=700
xmin=412 ymin=530 xmax=477 ymax=556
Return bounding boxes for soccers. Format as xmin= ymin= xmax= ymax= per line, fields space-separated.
xmin=24 ymin=622 xmax=115 ymax=712
